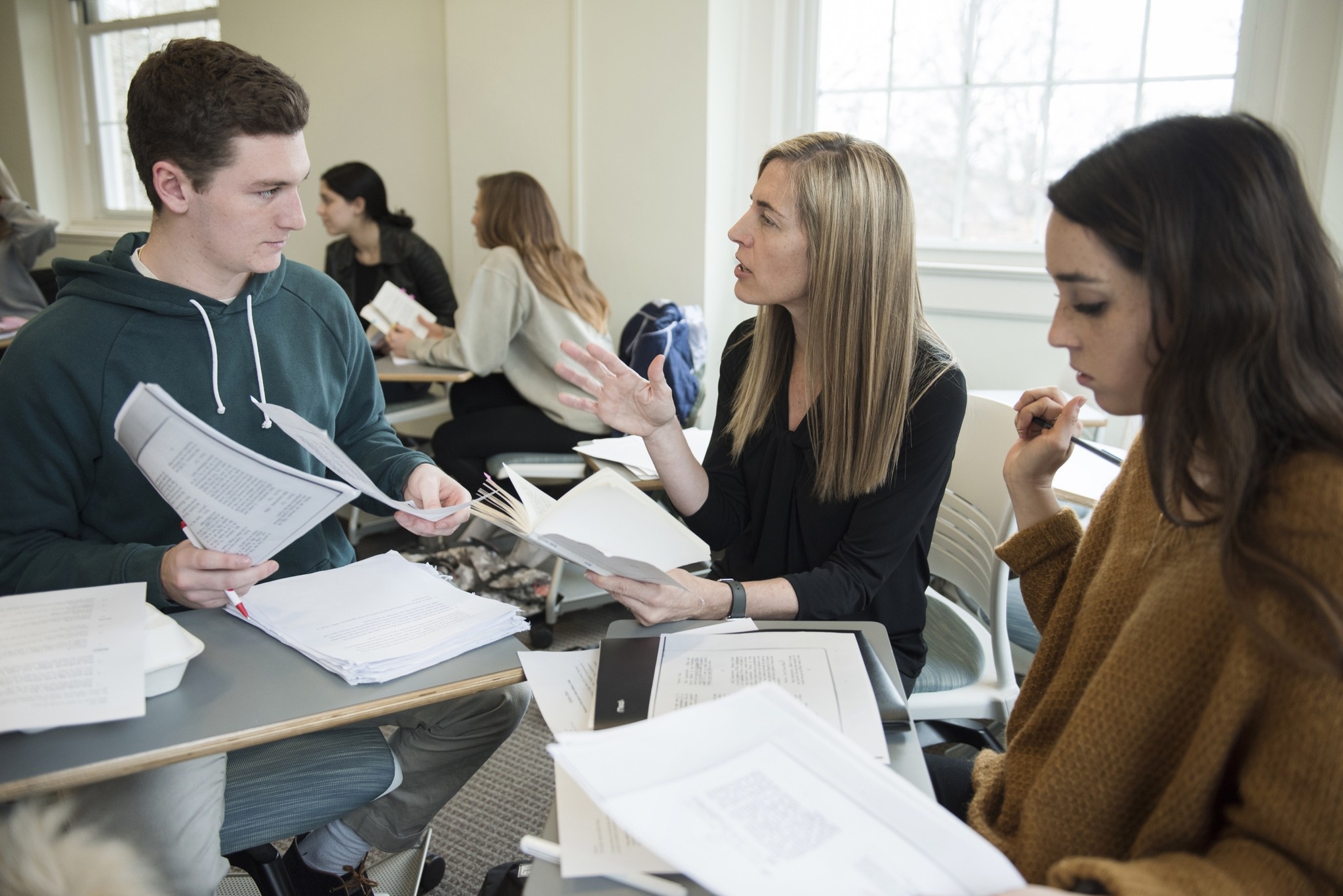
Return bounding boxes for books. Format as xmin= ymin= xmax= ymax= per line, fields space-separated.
xmin=360 ymin=281 xmax=437 ymax=340
xmin=470 ymin=460 xmax=711 ymax=607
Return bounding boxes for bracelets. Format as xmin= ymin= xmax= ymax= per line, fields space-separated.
xmin=717 ymin=579 xmax=746 ymax=620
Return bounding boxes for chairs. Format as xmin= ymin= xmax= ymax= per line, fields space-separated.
xmin=2 ymin=300 xmax=1126 ymax=896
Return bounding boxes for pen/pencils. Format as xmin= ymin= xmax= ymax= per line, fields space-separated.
xmin=371 ymin=304 xmax=394 ymax=329
xmin=1033 ymin=416 xmax=1122 ymax=466
xmin=180 ymin=520 xmax=251 ymax=622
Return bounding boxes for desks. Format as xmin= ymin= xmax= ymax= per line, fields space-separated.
xmin=0 ymin=610 xmax=532 ymax=802
xmin=578 ymin=440 xmax=664 ymax=490
xmin=374 ymin=357 xmax=472 ymax=382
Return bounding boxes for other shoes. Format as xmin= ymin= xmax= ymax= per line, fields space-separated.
xmin=276 ymin=831 xmax=378 ymax=896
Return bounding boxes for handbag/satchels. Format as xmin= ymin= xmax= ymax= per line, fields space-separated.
xmin=619 ymin=303 xmax=704 ymax=428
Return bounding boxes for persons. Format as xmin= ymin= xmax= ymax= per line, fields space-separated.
xmin=925 ymin=110 xmax=1343 ymax=896
xmin=387 ymin=171 xmax=606 ymax=502
xmin=0 ymin=158 xmax=59 ymax=312
xmin=0 ymin=39 xmax=533 ymax=896
xmin=317 ymin=161 xmax=458 ymax=401
xmin=555 ymin=132 xmax=969 ymax=703
